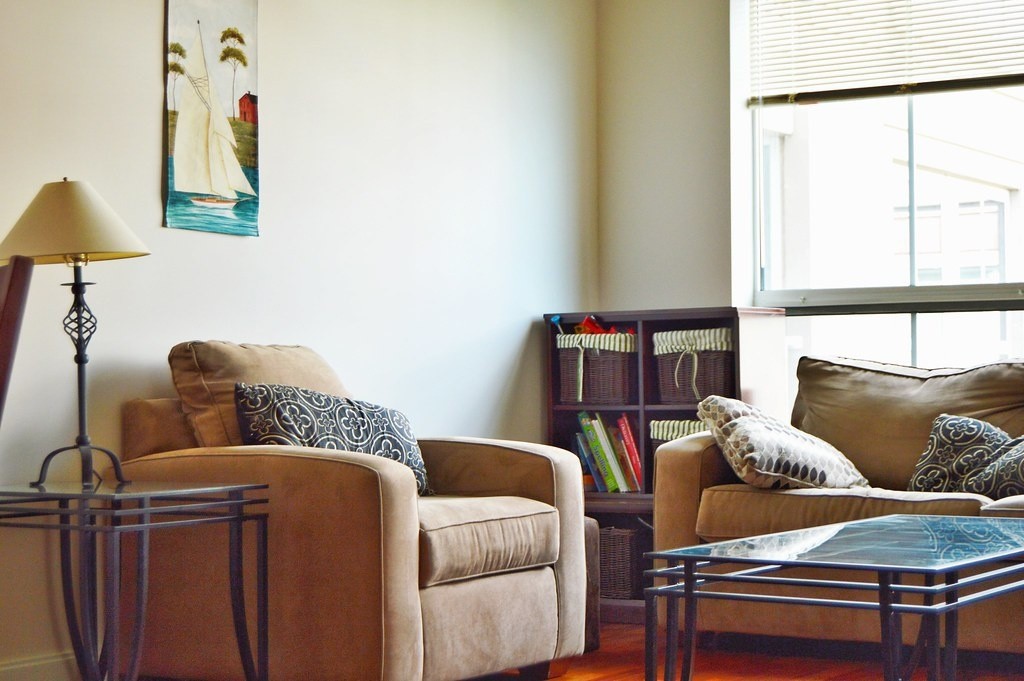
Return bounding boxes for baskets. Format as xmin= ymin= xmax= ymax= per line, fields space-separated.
xmin=556 ymin=331 xmax=638 ymax=406
xmin=650 ymin=419 xmax=712 ymax=466
xmin=599 ymin=524 xmax=653 ymax=598
xmin=652 ymin=330 xmax=736 ymax=404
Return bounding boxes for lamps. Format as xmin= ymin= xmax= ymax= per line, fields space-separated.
xmin=0 ymin=177 xmax=151 ymax=486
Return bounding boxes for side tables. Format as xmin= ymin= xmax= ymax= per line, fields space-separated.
xmin=0 ymin=482 xmax=271 ymax=681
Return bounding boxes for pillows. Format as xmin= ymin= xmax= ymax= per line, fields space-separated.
xmin=170 ymin=340 xmax=351 ymax=450
xmin=906 ymin=415 xmax=1024 ymax=496
xmin=696 ymin=394 xmax=871 ymax=491
xmin=232 ymin=380 xmax=427 ymax=497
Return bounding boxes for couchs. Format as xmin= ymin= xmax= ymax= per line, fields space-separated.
xmin=650 ymin=355 xmax=1024 ymax=661
xmin=103 ymin=398 xmax=587 ymax=681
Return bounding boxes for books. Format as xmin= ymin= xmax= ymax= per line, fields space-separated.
xmin=574 ymin=410 xmax=642 ymax=495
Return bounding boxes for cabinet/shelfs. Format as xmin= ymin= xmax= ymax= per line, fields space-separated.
xmin=541 ymin=305 xmax=740 ymax=624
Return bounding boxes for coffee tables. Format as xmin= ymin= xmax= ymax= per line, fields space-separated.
xmin=641 ymin=514 xmax=1024 ymax=681
